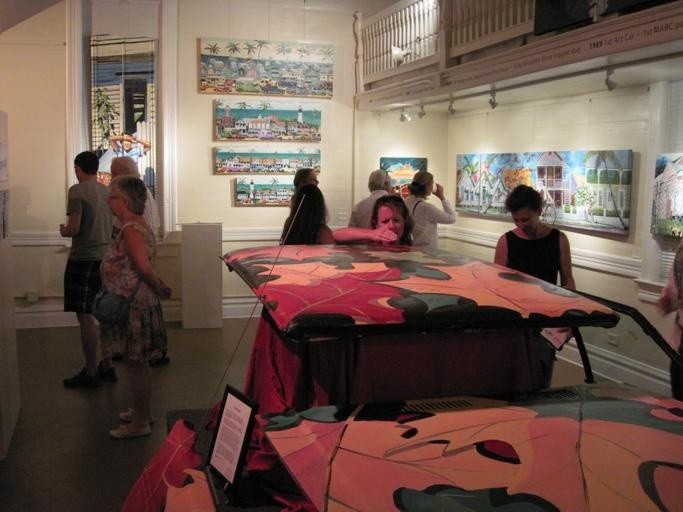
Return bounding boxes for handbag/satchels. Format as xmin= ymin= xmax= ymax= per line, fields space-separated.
xmin=92 ymin=288 xmax=133 ymax=326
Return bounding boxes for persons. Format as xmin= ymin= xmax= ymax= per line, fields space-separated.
xmin=59 ymin=152 xmax=118 ymax=390
xmin=659 ymin=240 xmax=682 ymax=401
xmin=404 ymin=172 xmax=457 ymax=247
xmin=100 ymin=176 xmax=173 ymax=440
xmin=493 ymin=185 xmax=578 ymax=390
xmin=111 ymin=156 xmax=170 ymax=367
xmin=347 ymin=169 xmax=392 ymax=228
xmin=538 ymin=180 xmax=552 ymax=216
xmin=279 ymin=185 xmax=333 ymax=245
xmin=331 ymin=195 xmax=416 ymax=246
xmin=293 ymin=168 xmax=319 ymax=189
xmin=108 ymin=133 xmax=152 ymax=168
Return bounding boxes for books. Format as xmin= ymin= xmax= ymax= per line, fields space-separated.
xmin=540 ymin=327 xmax=570 ymax=350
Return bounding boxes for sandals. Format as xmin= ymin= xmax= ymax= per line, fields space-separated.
xmin=110 ymin=408 xmax=157 ymax=441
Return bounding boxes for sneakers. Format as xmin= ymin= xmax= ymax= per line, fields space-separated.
xmin=63 ymin=360 xmax=118 ymax=390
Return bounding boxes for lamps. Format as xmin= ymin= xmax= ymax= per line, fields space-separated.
xmin=396 ymin=64 xmax=620 ymax=124
xmin=387 ymin=44 xmax=417 ymax=69
